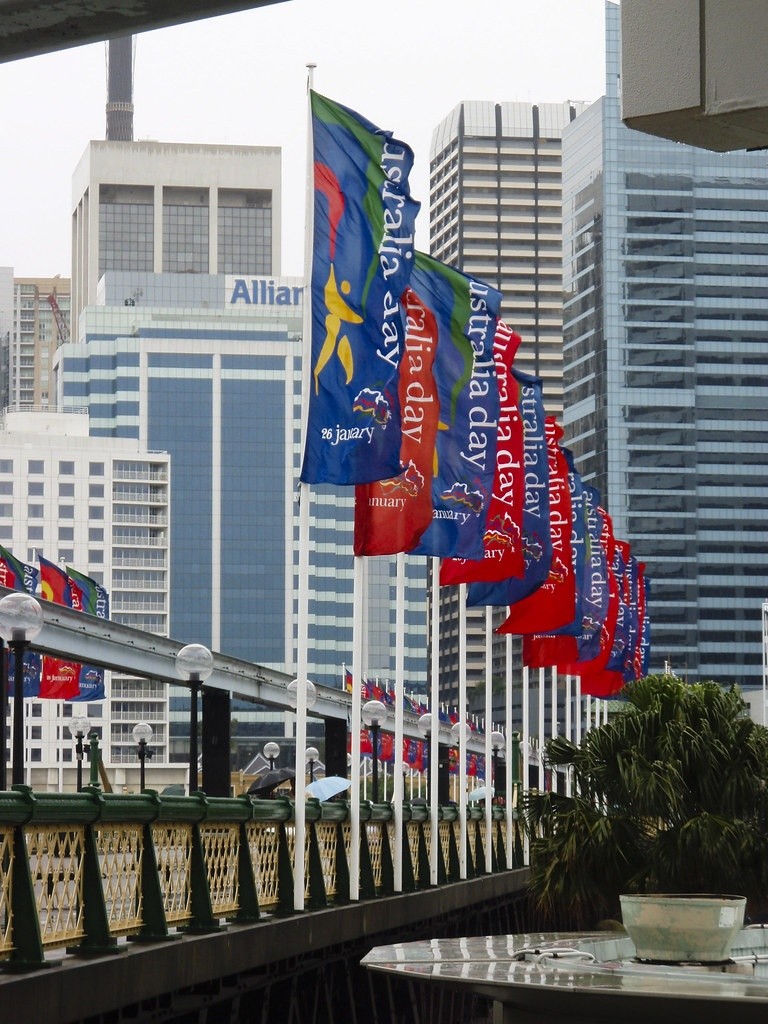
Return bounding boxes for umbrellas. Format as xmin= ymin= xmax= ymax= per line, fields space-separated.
xmin=247 ymin=769 xmax=296 ymax=797
xmin=305 ymin=777 xmax=351 ymax=802
xmin=470 ymin=786 xmax=494 ymax=800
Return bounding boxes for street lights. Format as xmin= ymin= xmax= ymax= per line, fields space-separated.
xmin=362 ymin=700 xmax=388 ymax=804
xmin=305 ymin=747 xmax=319 ymax=784
xmin=131 ymin=720 xmax=153 ymax=790
xmin=68 ymin=715 xmax=92 ymax=793
xmin=491 ymin=732 xmax=506 ymax=804
xmin=418 ymin=713 xmax=441 ymax=807
xmin=263 ymin=742 xmax=280 ymax=771
xmin=0 ymin=592 xmax=44 ymax=784
xmin=174 ymin=643 xmax=216 ymax=793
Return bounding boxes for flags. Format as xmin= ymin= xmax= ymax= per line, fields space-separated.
xmin=298 ymin=89 xmax=420 ymax=487
xmin=0 ymin=545 xmax=108 ymax=701
xmin=351 ymin=247 xmax=647 ymax=703
xmin=343 ymin=668 xmax=496 ymax=782
